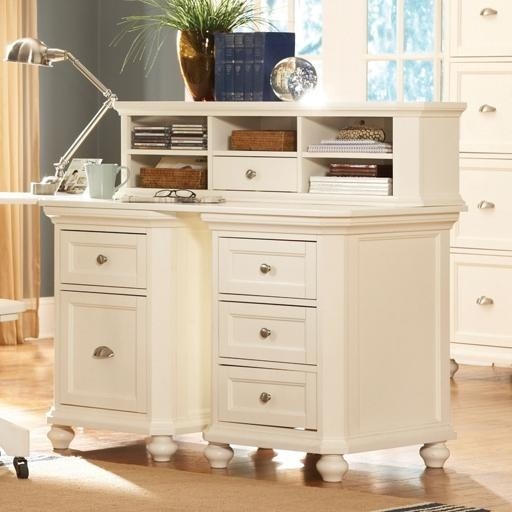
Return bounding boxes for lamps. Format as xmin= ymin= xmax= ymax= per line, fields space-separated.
xmin=3 ymin=37 xmax=118 ymax=195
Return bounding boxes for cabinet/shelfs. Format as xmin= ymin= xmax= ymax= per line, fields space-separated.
xmin=210 ymin=214 xmax=327 ymax=441
xmin=447 ymin=0 xmax=512 ymax=363
xmin=57 ymin=207 xmax=150 ymax=427
xmin=113 ymin=98 xmax=467 ymax=213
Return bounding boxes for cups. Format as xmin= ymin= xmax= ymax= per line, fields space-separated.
xmin=84 ymin=162 xmax=130 ymax=199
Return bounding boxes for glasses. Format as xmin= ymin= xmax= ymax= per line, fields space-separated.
xmin=154 ymin=190 xmax=196 ymax=198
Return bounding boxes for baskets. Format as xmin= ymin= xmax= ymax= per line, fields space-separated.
xmin=139 ymin=168 xmax=207 ymax=189
xmin=230 ymin=130 xmax=296 ymax=151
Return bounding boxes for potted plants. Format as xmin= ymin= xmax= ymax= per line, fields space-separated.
xmin=107 ymin=0 xmax=280 ymax=101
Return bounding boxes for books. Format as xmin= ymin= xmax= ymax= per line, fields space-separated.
xmin=308 ymin=138 xmax=392 ymax=153
xmin=132 ymin=124 xmax=207 ymax=150
xmin=309 ymin=175 xmax=393 ymax=196
